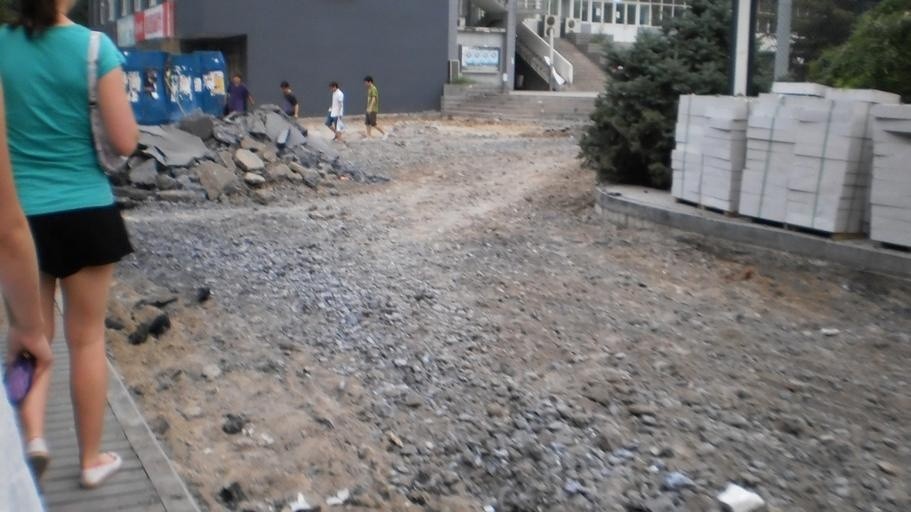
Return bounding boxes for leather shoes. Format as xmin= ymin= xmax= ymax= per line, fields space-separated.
xmin=79 ymin=451 xmax=122 ymax=490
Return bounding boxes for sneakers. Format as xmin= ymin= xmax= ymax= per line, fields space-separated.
xmin=24 ymin=439 xmax=50 ymax=498
xmin=362 ymin=132 xmax=389 ymax=141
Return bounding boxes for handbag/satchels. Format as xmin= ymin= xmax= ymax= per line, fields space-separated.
xmin=90 ymin=102 xmax=132 ymax=175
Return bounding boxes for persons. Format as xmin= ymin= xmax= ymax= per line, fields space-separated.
xmin=1 ymin=1 xmax=142 ymax=489
xmin=223 ymin=75 xmax=255 ymax=113
xmin=362 ymin=76 xmax=389 ymax=142
xmin=324 ymin=81 xmax=345 ymax=142
xmin=1 ymin=81 xmax=55 ymax=511
xmin=279 ymin=81 xmax=300 ymax=119
